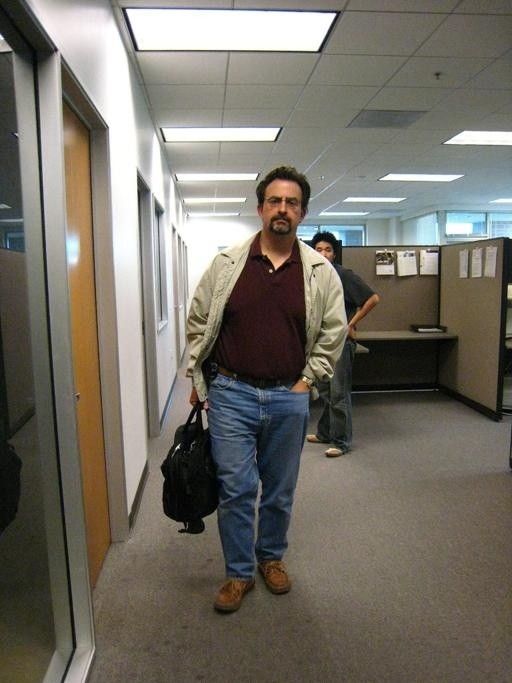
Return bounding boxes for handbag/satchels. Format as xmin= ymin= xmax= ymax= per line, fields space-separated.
xmin=160 ymin=400 xmax=220 ymax=526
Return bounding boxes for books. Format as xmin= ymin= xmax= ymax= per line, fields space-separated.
xmin=409 ymin=323 xmax=448 ymax=332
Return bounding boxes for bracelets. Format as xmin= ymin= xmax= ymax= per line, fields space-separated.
xmin=300 ymin=376 xmax=316 ymax=386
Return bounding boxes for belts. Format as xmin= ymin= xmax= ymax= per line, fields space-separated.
xmin=215 ymin=365 xmax=296 ymax=391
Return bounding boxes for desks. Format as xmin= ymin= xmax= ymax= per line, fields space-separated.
xmin=347 ymin=329 xmax=458 ymax=395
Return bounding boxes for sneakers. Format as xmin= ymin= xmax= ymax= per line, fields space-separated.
xmin=214 ymin=575 xmax=256 ymax=613
xmin=258 ymin=561 xmax=292 ymax=595
xmin=307 ymin=434 xmax=325 ymax=442
xmin=325 ymin=447 xmax=345 ymax=456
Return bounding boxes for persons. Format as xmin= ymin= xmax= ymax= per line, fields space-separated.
xmin=306 ymin=228 xmax=380 ymax=460
xmin=181 ymin=163 xmax=351 ymax=615
